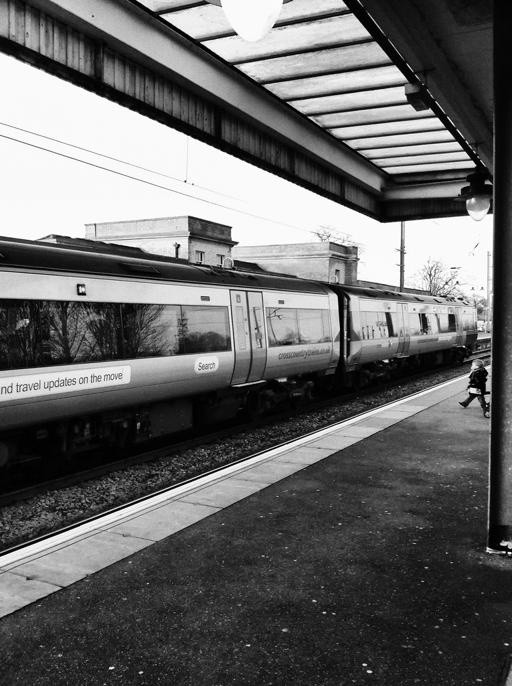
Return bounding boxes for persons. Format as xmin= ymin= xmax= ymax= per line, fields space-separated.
xmin=458 ymin=358 xmax=488 ymax=411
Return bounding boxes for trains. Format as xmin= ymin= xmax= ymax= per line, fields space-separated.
xmin=0 ymin=233 xmax=477 ymax=485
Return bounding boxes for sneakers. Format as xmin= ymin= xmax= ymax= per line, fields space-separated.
xmin=458 ymin=401 xmax=466 ymax=408
